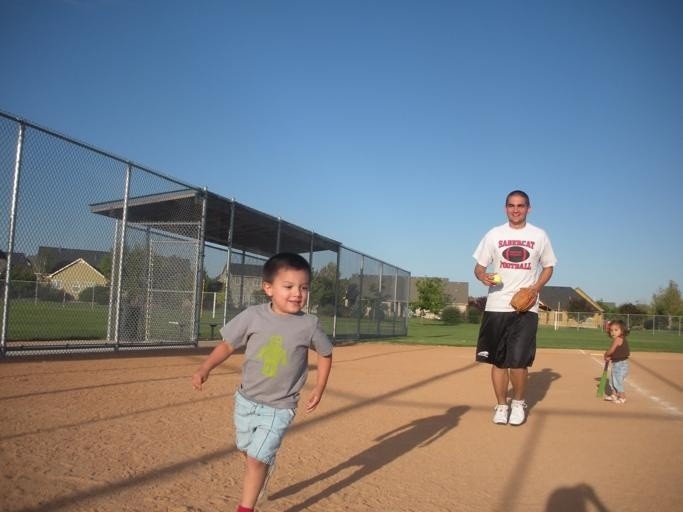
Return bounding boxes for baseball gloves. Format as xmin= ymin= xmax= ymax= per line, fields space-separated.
xmin=510 ymin=288 xmax=538 ymax=313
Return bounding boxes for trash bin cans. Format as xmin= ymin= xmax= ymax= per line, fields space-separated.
xmin=119 ymin=305 xmax=141 ymax=341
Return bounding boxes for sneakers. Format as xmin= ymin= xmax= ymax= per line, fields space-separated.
xmin=493 ymin=404 xmax=509 ymax=425
xmin=509 ymin=400 xmax=526 ymax=425
xmin=605 ymin=394 xmax=626 ymax=404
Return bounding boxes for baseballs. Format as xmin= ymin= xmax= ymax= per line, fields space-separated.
xmin=491 ymin=275 xmax=502 ymax=285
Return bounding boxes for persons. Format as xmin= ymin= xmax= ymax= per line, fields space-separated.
xmin=603 ymin=319 xmax=632 ymax=405
xmin=472 ymin=190 xmax=557 ymax=426
xmin=190 ymin=251 xmax=333 ymax=511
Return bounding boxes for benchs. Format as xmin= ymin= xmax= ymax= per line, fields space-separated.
xmin=169 ymin=320 xmax=224 ymax=340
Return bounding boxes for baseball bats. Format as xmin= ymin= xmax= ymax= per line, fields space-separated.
xmin=596 ymin=361 xmax=611 ymax=397
